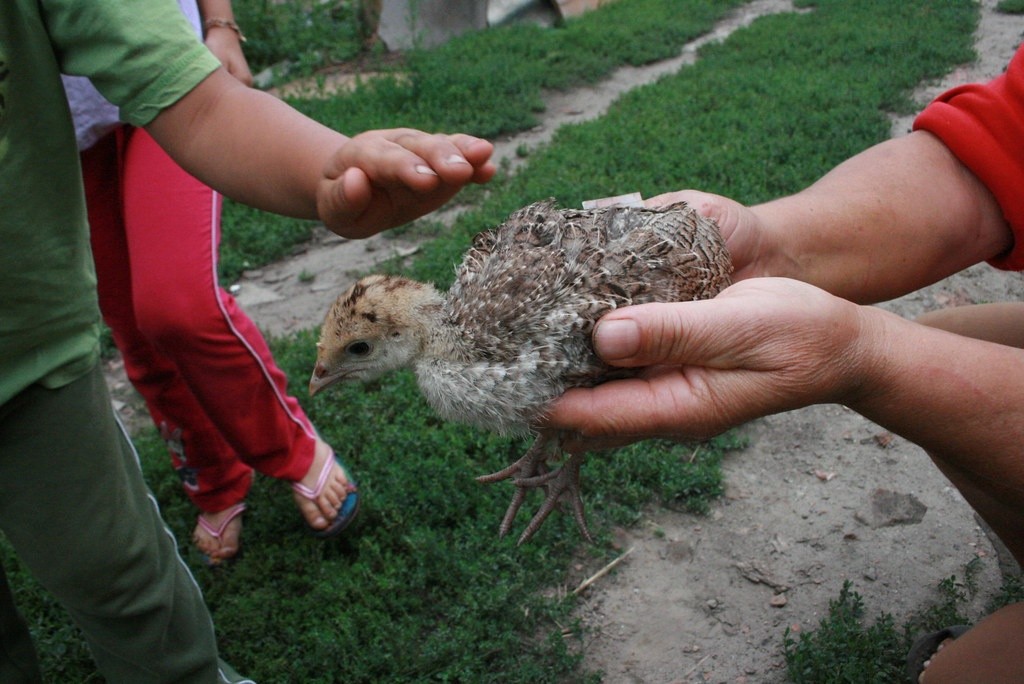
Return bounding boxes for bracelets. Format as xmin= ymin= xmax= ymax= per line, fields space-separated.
xmin=204 ymin=17 xmax=245 ymax=42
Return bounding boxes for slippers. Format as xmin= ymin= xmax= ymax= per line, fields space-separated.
xmin=190 ymin=503 xmax=248 ymax=566
xmin=288 ymin=423 xmax=360 ymax=539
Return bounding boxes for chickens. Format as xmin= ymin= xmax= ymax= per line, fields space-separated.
xmin=308 ymin=197 xmax=735 ymax=547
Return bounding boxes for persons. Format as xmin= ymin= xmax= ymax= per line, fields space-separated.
xmin=59 ymin=0 xmax=361 ymax=566
xmin=532 ymin=40 xmax=1022 ymax=682
xmin=0 ymin=0 xmax=494 ymax=684
